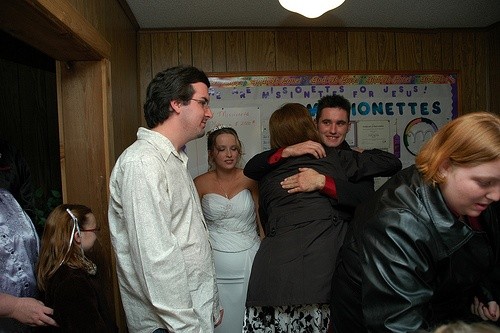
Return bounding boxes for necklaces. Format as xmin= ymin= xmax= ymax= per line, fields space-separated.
xmin=216 ymin=169 xmax=236 ymax=198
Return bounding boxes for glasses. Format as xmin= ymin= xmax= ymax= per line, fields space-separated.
xmin=179 ymin=98 xmax=210 ymax=110
xmin=80 ymin=226 xmax=103 ymax=234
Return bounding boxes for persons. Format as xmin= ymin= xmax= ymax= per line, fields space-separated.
xmin=192 ymin=125 xmax=265 ymax=333
xmin=242 ymin=95 xmax=500 ymax=333
xmin=108 ymin=65 xmax=225 ymax=333
xmin=0 ymin=168 xmax=120 ymax=333
